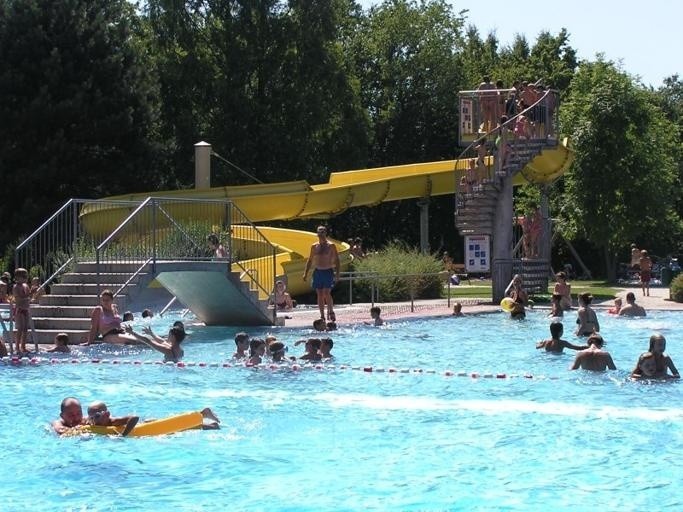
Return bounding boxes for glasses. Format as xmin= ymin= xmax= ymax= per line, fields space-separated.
xmin=91 ymin=412 xmax=103 ymax=422
xmin=283 ymin=344 xmax=289 ymax=353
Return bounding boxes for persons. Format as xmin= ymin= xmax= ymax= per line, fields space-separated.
xmin=363 ymin=306 xmax=383 ymax=327
xmin=0 ymin=268 xmax=189 ymax=366
xmin=503 ymin=243 xmax=683 ymax=383
xmin=228 ymin=317 xmax=340 ymax=366
xmin=346 ymin=236 xmax=367 ymax=262
xmin=83 ymin=400 xmax=224 ymax=438
xmin=266 ymin=280 xmax=294 ymax=313
xmin=457 ymin=73 xmax=555 ymax=257
xmin=54 ymin=395 xmax=91 ymax=436
xmin=440 ymin=251 xmax=457 ymax=284
xmin=450 ymin=303 xmax=466 ymax=318
xmin=304 ymin=225 xmax=341 ymax=322
xmin=207 ymin=234 xmax=231 ymax=261
